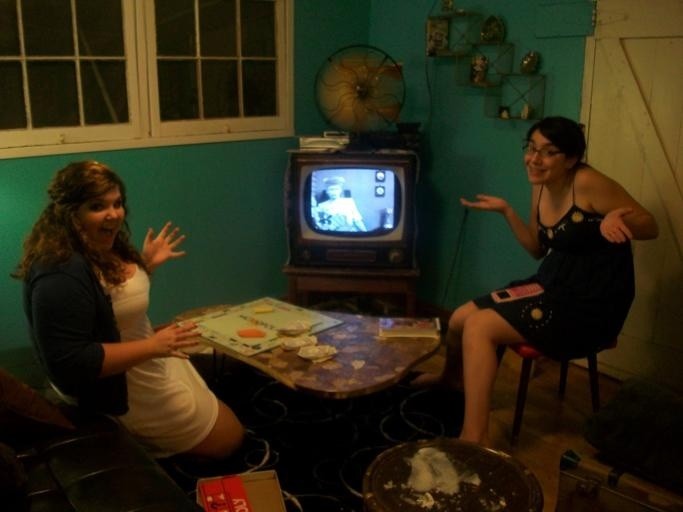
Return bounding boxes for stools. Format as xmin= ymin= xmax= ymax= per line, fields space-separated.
xmin=495 ymin=335 xmax=618 ymax=446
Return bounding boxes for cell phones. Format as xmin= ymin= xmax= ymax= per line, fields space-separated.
xmin=490 ymin=283 xmax=545 ymax=304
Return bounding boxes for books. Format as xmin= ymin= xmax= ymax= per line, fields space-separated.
xmin=377 ymin=315 xmax=441 ymax=339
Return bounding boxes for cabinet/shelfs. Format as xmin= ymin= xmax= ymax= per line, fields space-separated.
xmin=425 ymin=11 xmax=546 ymax=121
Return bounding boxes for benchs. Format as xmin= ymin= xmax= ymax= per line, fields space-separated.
xmin=20 ymin=377 xmax=201 ymax=510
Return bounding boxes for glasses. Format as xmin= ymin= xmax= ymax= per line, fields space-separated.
xmin=519 ymin=142 xmax=564 ymax=158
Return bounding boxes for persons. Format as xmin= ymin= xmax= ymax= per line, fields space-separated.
xmin=7 ymin=158 xmax=245 ymax=458
xmin=314 ymin=176 xmax=371 ymax=232
xmin=444 ymin=115 xmax=658 ymax=448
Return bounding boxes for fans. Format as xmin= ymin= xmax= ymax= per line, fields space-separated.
xmin=313 ymin=42 xmax=407 ymax=150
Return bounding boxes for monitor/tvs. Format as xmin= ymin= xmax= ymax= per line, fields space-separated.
xmin=291 ymin=156 xmax=412 ymax=268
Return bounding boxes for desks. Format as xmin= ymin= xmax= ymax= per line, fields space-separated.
xmin=280 ymin=260 xmax=421 ymax=319
xmin=170 ymin=295 xmax=445 ymax=452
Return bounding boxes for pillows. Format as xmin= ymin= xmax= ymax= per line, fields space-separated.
xmin=0 ymin=363 xmax=79 ymax=432
